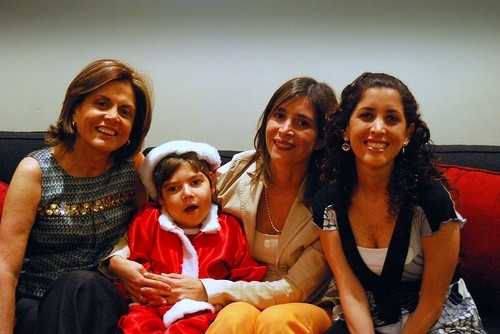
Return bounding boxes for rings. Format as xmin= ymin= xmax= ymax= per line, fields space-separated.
xmin=139 ymin=294 xmax=144 ymax=302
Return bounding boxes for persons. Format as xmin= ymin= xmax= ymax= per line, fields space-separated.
xmin=0 ymin=59 xmax=153 ymax=334
xmin=312 ymin=73 xmax=486 ymax=334
xmin=98 ymin=77 xmax=342 ymax=334
xmin=114 ymin=140 xmax=268 ymax=334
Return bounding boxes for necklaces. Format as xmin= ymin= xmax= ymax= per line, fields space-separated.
xmin=264 ymin=188 xmax=282 ymax=233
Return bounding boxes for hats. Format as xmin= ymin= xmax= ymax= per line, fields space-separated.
xmin=141 ymin=140 xmax=221 ymax=200
